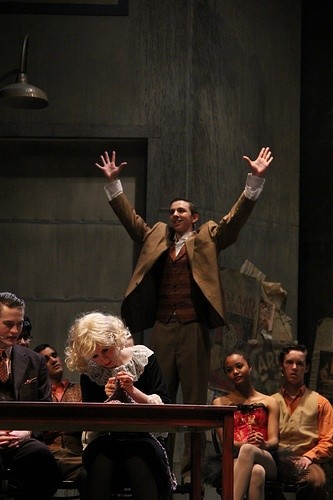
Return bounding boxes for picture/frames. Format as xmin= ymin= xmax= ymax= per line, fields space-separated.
xmin=0 ymin=0 xmax=129 ymax=16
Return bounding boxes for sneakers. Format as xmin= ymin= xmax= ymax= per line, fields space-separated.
xmin=181 ymin=475 xmax=192 ymax=492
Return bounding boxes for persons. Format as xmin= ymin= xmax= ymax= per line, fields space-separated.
xmin=63 ymin=310 xmax=178 ymax=500
xmin=0 ymin=292 xmax=57 ymax=500
xmin=94 ymin=147 xmax=274 ymax=500
xmin=33 ymin=343 xmax=82 ymax=403
xmin=18 ymin=318 xmax=31 ymax=347
xmin=267 ymin=343 xmax=333 ymax=500
xmin=211 ymin=350 xmax=281 ymax=500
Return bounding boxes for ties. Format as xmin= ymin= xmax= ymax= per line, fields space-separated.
xmin=0 ymin=348 xmax=10 ymax=385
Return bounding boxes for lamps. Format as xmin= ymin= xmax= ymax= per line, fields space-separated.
xmin=0 ymin=34 xmax=49 ymax=109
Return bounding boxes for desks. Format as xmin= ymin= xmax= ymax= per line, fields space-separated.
xmin=0 ymin=401 xmax=237 ymax=499
